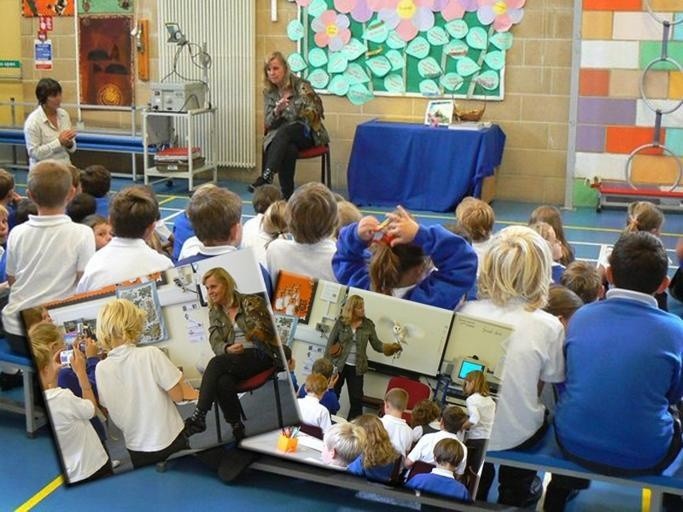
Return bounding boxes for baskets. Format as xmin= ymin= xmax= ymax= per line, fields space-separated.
xmin=452 ymin=80 xmax=486 ymax=121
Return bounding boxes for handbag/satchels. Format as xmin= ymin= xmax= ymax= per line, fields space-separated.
xmin=328 ymin=344 xmax=343 ymax=357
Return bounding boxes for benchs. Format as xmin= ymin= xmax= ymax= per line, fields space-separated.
xmin=0 ymin=342 xmax=46 ymax=439
xmin=596 ymin=182 xmax=683 ymax=212
xmin=0 ymin=97 xmax=165 ymax=181
xmin=239 ymin=424 xmax=683 ymax=512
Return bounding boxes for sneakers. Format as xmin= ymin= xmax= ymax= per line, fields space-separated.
xmin=248 ymin=175 xmax=273 ymax=192
xmin=183 ymin=417 xmax=205 ymax=437
xmin=232 ymin=422 xmax=244 ymax=439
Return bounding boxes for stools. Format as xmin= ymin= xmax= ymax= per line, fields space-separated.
xmin=347 ymin=396 xmax=384 ymax=421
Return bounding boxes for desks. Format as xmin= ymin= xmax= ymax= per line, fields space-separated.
xmin=348 ymin=119 xmax=506 ymax=207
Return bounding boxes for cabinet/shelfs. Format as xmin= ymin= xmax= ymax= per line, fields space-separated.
xmin=142 ymin=110 xmax=218 ymax=194
xmin=433 ymin=376 xmax=497 ymax=411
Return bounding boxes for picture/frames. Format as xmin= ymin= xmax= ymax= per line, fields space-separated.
xmin=424 ymin=99 xmax=454 ymax=126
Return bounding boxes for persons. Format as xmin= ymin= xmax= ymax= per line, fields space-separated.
xmin=1 ymin=160 xmax=683 ymax=511
xmin=245 ymin=50 xmax=331 ymax=203
xmin=23 ymin=76 xmax=79 ymax=176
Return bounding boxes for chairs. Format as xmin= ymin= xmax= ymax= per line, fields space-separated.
xmin=406 ymin=462 xmax=459 ymax=481
xmin=378 ymin=375 xmax=430 ymax=423
xmin=261 ymin=126 xmax=332 ymax=189
xmin=391 ymin=454 xmax=403 ymax=480
xmin=214 ymin=292 xmax=289 ymax=441
xmin=295 ymin=421 xmax=323 ymax=439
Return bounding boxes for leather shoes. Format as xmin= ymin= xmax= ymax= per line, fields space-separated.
xmin=498 ymin=476 xmax=542 ymax=507
